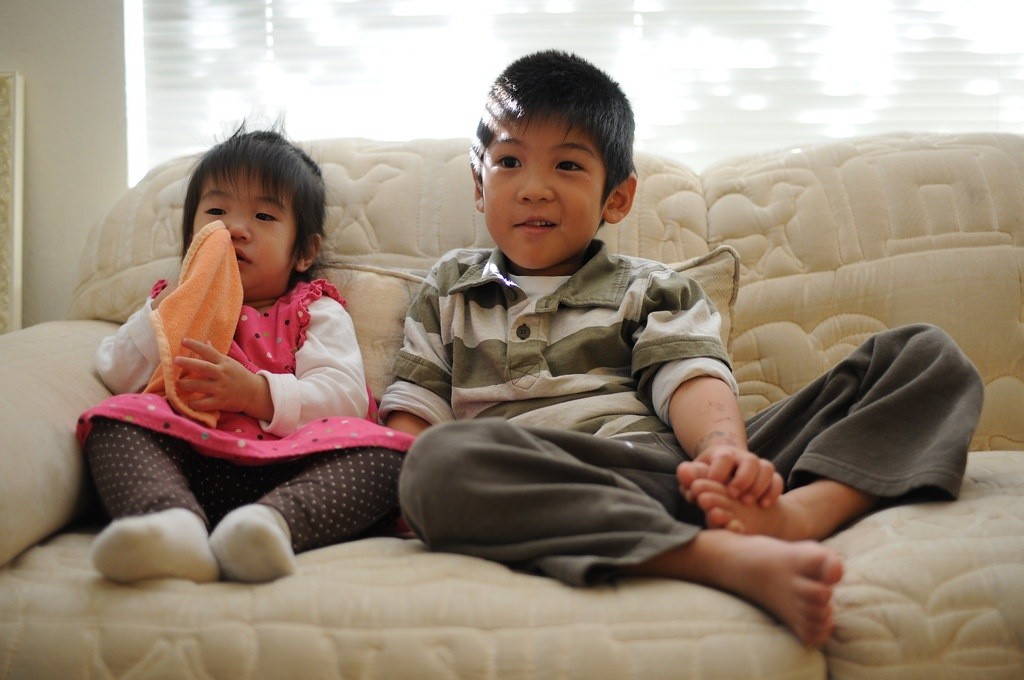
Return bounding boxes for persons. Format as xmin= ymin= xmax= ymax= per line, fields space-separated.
xmin=77 ymin=129 xmax=416 ymax=586
xmin=379 ymin=49 xmax=984 ymax=645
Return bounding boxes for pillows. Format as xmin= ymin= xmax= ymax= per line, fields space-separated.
xmin=319 ymin=244 xmax=740 ymax=402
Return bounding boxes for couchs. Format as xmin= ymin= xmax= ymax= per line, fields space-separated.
xmin=0 ymin=131 xmax=1023 ymax=679
